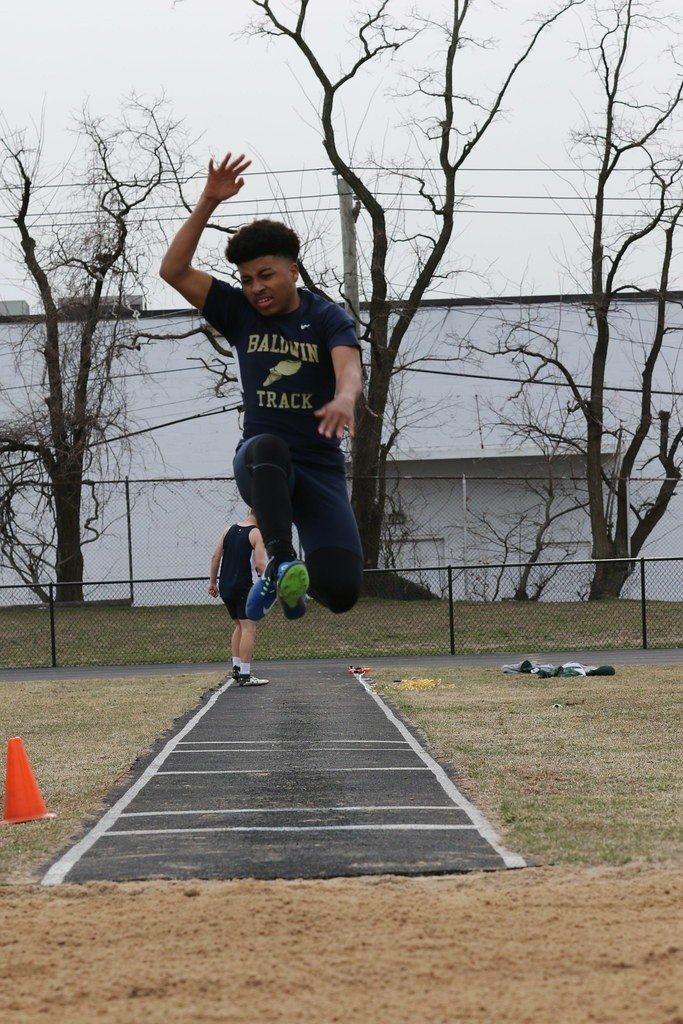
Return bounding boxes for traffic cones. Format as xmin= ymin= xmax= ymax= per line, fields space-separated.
xmin=0 ymin=737 xmax=59 ymax=826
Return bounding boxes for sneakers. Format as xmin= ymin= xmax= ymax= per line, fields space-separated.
xmin=232 ymin=666 xmax=240 ymax=681
xmin=246 ymin=556 xmax=279 ymax=621
xmin=239 ymin=676 xmax=269 ymax=686
xmin=275 ymin=560 xmax=309 ymax=619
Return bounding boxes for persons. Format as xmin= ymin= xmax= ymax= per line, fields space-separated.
xmin=160 ymin=153 xmax=365 ymax=618
xmin=208 ymin=511 xmax=269 ymax=686
